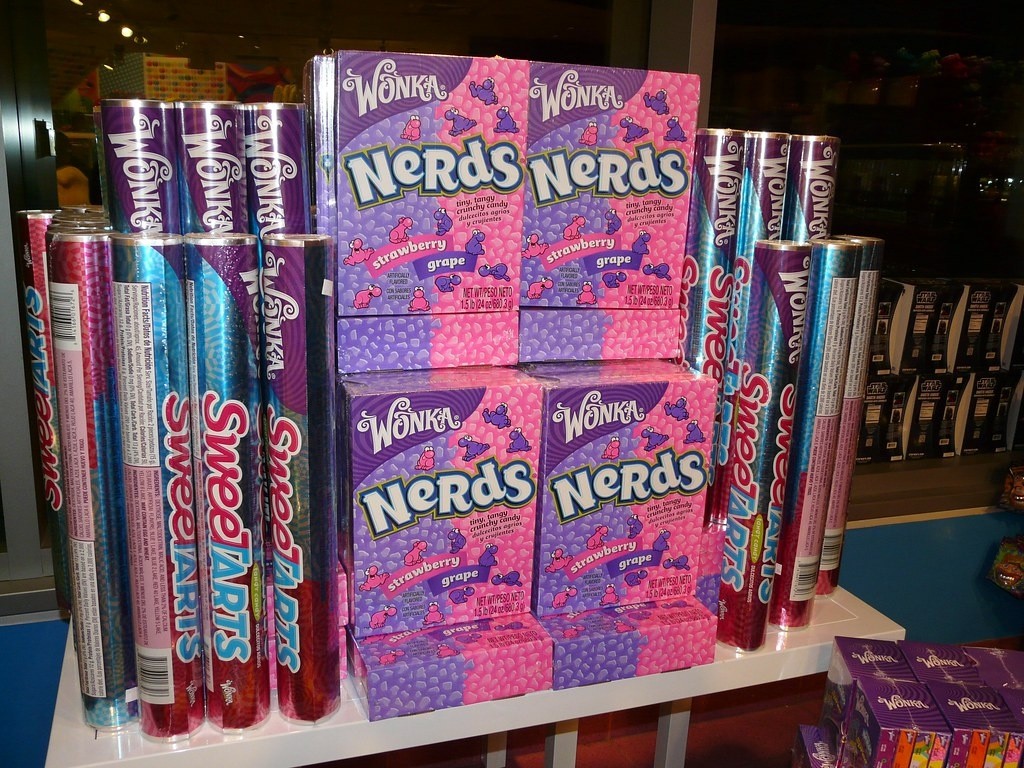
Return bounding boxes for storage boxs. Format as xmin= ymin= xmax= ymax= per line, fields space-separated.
xmin=302 ymin=48 xmax=717 ymax=721
xmin=852 ymin=276 xmax=1023 ymax=463
xmin=795 ymin=636 xmax=1022 ymax=767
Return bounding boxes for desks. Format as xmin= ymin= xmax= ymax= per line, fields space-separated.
xmin=42 ymin=585 xmax=907 ymax=768
